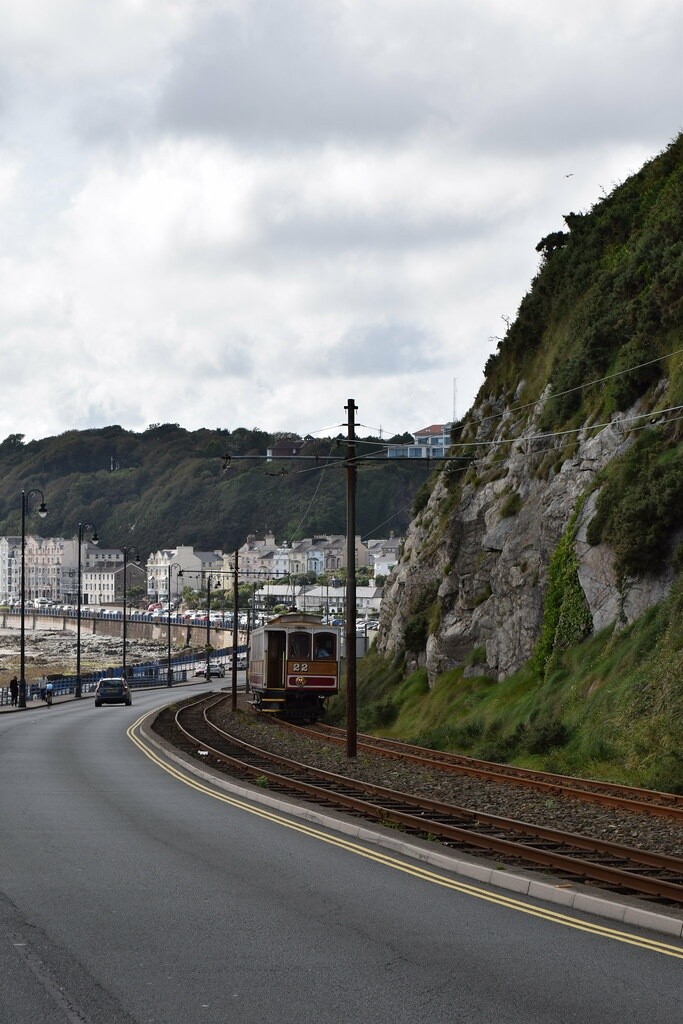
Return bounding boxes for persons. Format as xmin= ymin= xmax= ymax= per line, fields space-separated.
xmin=10 ymin=676 xmax=18 ymax=707
xmin=40 ymin=674 xmax=53 ymax=705
xmin=307 ymin=637 xmax=331 ymax=659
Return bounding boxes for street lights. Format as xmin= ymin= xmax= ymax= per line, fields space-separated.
xmin=168 ymin=562 xmax=184 ymax=686
xmin=206 ymin=573 xmax=221 ymax=680
xmin=75 ymin=519 xmax=99 ymax=677
xmin=17 ymin=488 xmax=48 ymax=706
xmin=121 ymin=545 xmax=141 ymax=680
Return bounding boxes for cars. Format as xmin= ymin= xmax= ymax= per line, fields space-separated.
xmin=95 ymin=677 xmax=132 ymax=708
xmin=194 ymin=666 xmax=204 ymax=677
xmin=0 ymin=595 xmax=382 ymax=634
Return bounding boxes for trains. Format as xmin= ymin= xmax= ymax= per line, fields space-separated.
xmin=246 ymin=613 xmax=340 ymax=725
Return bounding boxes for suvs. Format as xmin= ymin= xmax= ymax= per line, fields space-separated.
xmin=229 ymin=656 xmax=244 ymax=670
xmin=204 ymin=662 xmax=225 ymax=678
